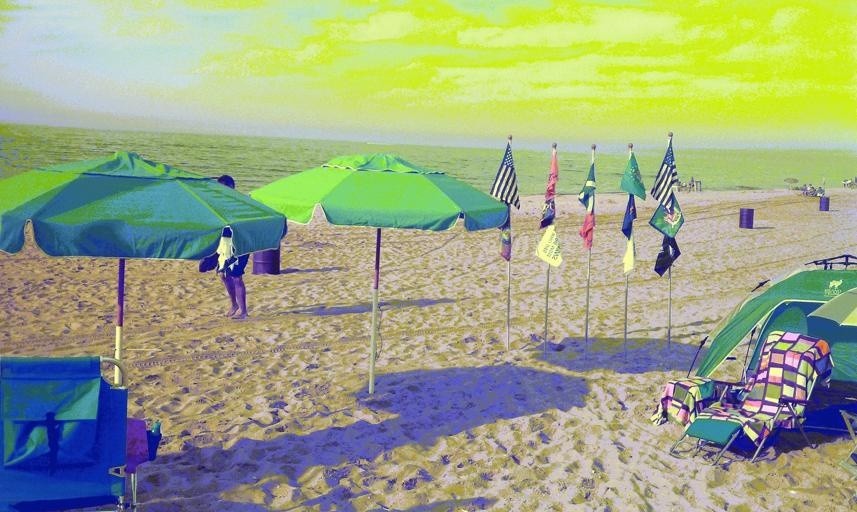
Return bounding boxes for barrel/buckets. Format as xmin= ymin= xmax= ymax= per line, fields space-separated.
xmin=740 ymin=207 xmax=754 ymax=229
xmin=251 ymin=244 xmax=281 ymax=275
xmin=820 ymin=196 xmax=830 ymax=211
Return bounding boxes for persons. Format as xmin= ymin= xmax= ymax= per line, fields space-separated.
xmin=214 ymin=174 xmax=250 ymax=320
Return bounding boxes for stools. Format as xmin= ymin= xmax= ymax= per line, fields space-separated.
xmin=650 ymin=372 xmax=715 ymax=430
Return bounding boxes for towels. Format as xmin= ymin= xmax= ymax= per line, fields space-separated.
xmin=650 ymin=376 xmax=732 ymax=425
xmin=694 ymin=332 xmax=834 ymax=445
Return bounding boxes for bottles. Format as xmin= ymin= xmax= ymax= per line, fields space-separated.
xmin=150 ymin=421 xmax=161 ymax=435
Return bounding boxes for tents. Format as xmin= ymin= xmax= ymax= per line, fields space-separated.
xmin=693 ymin=264 xmax=857 ymax=437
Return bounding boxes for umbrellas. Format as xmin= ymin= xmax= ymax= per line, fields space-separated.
xmin=250 ymin=151 xmax=510 ymax=395
xmin=0 ymin=149 xmax=289 ymax=389
xmin=809 ymin=282 xmax=857 ymax=328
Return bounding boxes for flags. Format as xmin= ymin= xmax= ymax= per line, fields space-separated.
xmin=620 ymin=151 xmax=646 ymax=277
xmin=579 ymin=158 xmax=599 ymax=250
xmin=490 ymin=142 xmax=520 ymax=260
xmin=650 ymin=139 xmax=685 ymax=277
xmin=535 ymin=151 xmax=563 ymax=267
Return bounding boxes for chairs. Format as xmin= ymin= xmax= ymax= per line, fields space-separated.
xmin=0 ymin=352 xmax=128 ymax=511
xmin=673 ymin=331 xmax=835 ymax=468
xmin=95 ymin=357 xmax=163 ymax=511
